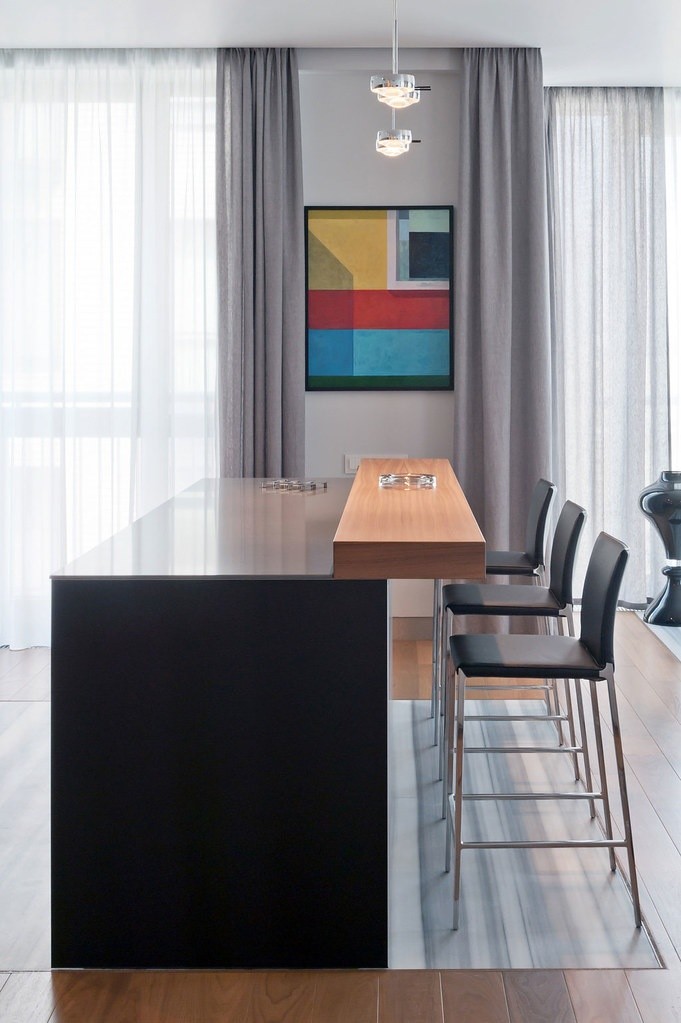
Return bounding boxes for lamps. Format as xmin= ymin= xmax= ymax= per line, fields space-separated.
xmin=370 ymin=0 xmax=424 ymax=157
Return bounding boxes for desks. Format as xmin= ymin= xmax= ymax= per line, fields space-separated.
xmin=52 ymin=459 xmax=487 ymax=970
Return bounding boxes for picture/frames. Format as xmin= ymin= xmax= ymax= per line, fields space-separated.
xmin=303 ymin=205 xmax=456 ymax=392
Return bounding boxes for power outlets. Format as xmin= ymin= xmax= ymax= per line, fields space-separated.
xmin=345 ymin=454 xmax=409 ymax=474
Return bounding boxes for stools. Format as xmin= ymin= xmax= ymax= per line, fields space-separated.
xmin=429 ymin=478 xmax=557 ymax=747
xmin=437 ymin=500 xmax=603 ymax=819
xmin=443 ymin=531 xmax=642 ymax=931
